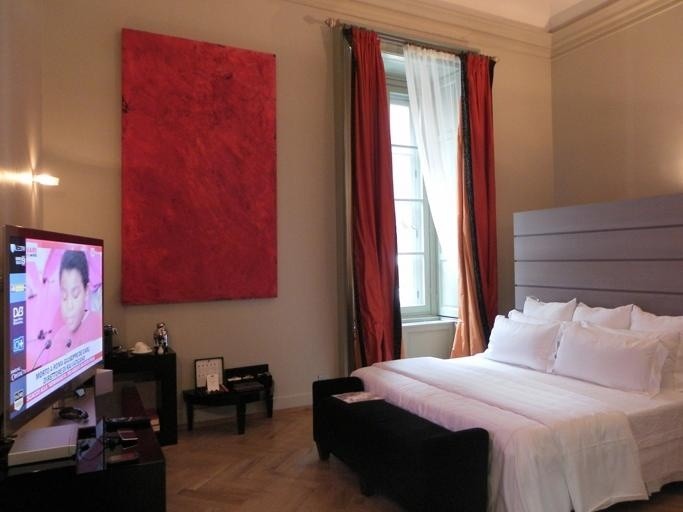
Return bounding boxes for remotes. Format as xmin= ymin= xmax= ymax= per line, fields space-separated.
xmin=108 ymin=452 xmax=140 ymax=463
xmin=106 ymin=414 xmax=150 ymax=425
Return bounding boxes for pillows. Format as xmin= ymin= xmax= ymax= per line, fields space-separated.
xmin=484 ymin=295 xmax=683 ymax=398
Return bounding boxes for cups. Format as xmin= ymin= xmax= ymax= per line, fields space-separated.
xmin=135 ymin=341 xmax=147 ymax=352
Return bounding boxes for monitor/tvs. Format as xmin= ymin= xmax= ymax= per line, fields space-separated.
xmin=1 ymin=225 xmax=104 ymax=435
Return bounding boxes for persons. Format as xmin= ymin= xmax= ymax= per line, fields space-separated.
xmin=44 ymin=250 xmax=102 ymax=364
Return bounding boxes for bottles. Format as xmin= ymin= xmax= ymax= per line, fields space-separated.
xmin=153 ymin=322 xmax=168 ymax=348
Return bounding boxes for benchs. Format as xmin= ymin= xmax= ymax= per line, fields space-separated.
xmin=312 ymin=376 xmax=489 ymax=512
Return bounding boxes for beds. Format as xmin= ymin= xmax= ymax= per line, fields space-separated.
xmin=350 ymin=193 xmax=683 ymax=512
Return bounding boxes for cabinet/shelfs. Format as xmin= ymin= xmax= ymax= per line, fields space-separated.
xmin=104 ymin=347 xmax=178 ymax=447
xmin=0 ymin=380 xmax=166 ymax=512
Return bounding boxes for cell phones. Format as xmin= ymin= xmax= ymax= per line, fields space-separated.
xmin=119 ymin=428 xmax=139 ymax=442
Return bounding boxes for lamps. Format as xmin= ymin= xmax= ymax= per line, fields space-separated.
xmin=33 ymin=175 xmax=59 ymax=187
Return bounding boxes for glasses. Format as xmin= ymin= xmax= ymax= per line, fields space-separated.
xmin=59 ymin=407 xmax=89 ymax=420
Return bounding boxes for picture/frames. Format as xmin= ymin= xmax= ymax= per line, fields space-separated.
xmin=194 ymin=357 xmax=224 ymax=390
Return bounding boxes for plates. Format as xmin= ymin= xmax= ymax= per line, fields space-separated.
xmin=132 ymin=350 xmax=153 ymax=354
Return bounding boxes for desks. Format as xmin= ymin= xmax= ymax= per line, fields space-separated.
xmin=183 ymin=364 xmax=274 ymax=435
